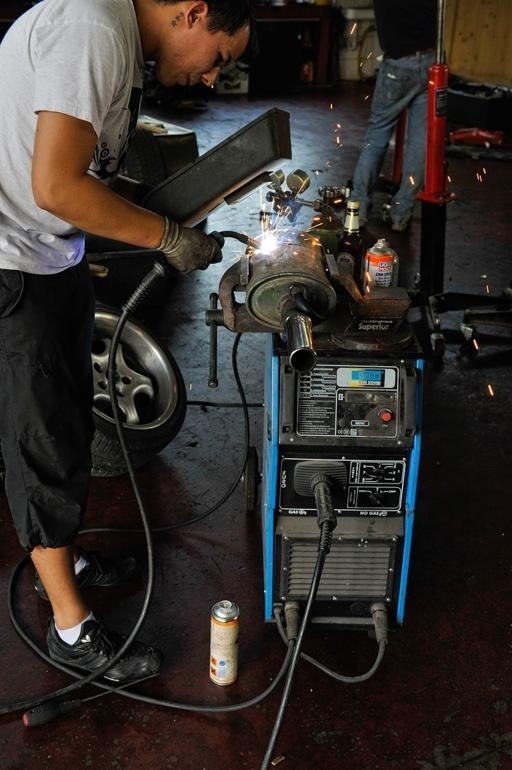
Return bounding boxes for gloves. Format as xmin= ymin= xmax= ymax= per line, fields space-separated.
xmin=153 ymin=215 xmax=224 ymax=273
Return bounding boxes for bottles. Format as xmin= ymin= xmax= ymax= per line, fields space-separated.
xmin=337 ymin=200 xmax=364 ymax=294
xmin=364 ymin=238 xmax=402 ymax=290
xmin=208 ymin=601 xmax=240 ymax=689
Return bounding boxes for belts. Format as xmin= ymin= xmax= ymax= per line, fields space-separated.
xmin=403 ymin=47 xmax=437 ymax=55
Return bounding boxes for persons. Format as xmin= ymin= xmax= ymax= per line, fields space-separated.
xmin=1 ymin=1 xmax=251 ymax=683
xmin=346 ymin=1 xmax=445 ymax=233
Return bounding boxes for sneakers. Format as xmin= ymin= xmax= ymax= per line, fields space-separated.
xmin=341 ymin=204 xmax=408 ymax=233
xmin=45 ymin=614 xmax=165 ymax=684
xmin=33 ymin=551 xmax=125 ymax=602
